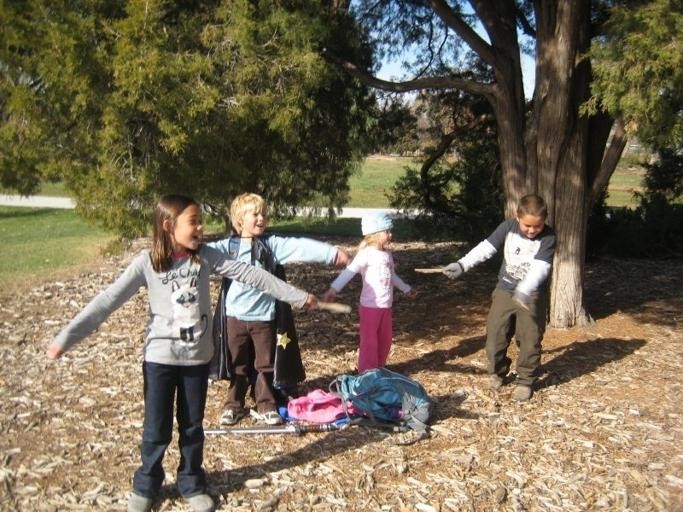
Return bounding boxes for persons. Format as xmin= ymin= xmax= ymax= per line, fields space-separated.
xmin=321 ymin=211 xmax=420 ymax=374
xmin=44 ymin=194 xmax=323 ymax=510
xmin=441 ymin=194 xmax=557 ymax=401
xmin=199 ymin=191 xmax=354 ymax=426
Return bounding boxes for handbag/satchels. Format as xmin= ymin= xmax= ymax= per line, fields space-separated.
xmin=287 ymin=388 xmax=363 ymax=423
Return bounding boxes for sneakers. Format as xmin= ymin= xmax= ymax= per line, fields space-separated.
xmin=489 ymin=356 xmax=512 ymax=390
xmin=126 ymin=491 xmax=153 ymax=512
xmin=511 ymin=383 xmax=532 ymax=402
xmin=183 ymin=493 xmax=215 ymax=512
xmin=220 ymin=407 xmax=245 ymax=425
xmin=253 ymin=404 xmax=284 ymax=425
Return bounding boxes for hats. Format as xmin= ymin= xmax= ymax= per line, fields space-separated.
xmin=361 ymin=210 xmax=395 ymax=236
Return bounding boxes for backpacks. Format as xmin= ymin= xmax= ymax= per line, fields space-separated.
xmin=328 ymin=367 xmax=438 ymax=445
